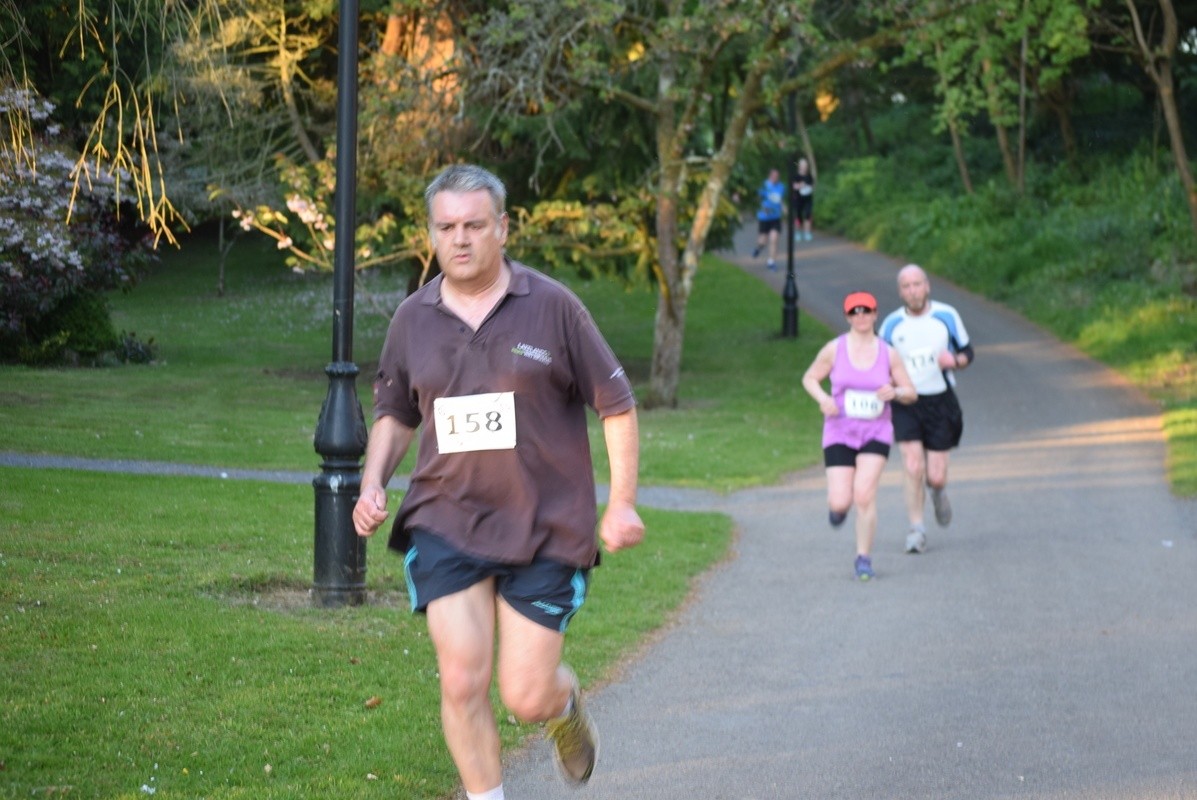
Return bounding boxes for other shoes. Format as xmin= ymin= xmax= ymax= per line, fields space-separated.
xmin=752 ymin=248 xmax=759 ymax=257
xmin=805 ymin=232 xmax=813 ymax=241
xmin=794 ymin=231 xmax=801 ymax=241
xmin=766 ymin=261 xmax=777 ymax=272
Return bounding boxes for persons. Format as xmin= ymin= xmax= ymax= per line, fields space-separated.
xmin=802 ymin=291 xmax=918 ymax=580
xmin=878 ymin=265 xmax=975 ymax=553
xmin=352 ymin=167 xmax=646 ymax=800
xmin=791 ymin=157 xmax=815 ymax=241
xmin=751 ymin=169 xmax=787 ymax=268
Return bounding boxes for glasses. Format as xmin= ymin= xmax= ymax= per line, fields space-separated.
xmin=848 ymin=306 xmax=874 ymax=315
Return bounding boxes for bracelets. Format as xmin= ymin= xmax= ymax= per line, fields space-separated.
xmin=953 ymin=354 xmax=959 ymax=367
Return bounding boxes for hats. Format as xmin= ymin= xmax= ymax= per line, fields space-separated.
xmin=843 ymin=293 xmax=878 ymax=314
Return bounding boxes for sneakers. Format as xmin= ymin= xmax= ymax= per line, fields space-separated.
xmin=855 ymin=556 xmax=873 ymax=580
xmin=536 ymin=664 xmax=599 ymax=788
xmin=829 ymin=511 xmax=846 ymax=526
xmin=904 ymin=525 xmax=927 ymax=552
xmin=929 ymin=487 xmax=954 ymax=526
